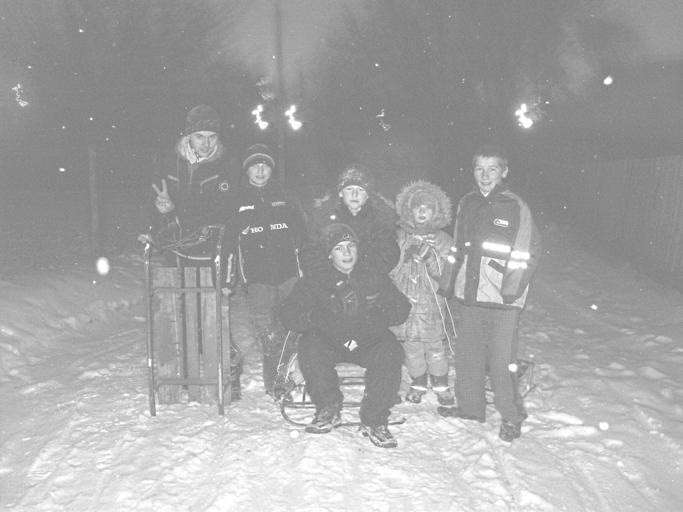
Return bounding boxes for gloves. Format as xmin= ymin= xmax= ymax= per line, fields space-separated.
xmin=408 ymin=245 xmax=419 ymax=263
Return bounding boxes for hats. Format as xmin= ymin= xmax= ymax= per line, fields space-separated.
xmin=324 ymin=222 xmax=359 ymax=255
xmin=243 ymin=143 xmax=275 ymax=174
xmin=186 ymin=105 xmax=222 ymax=133
xmin=337 ymin=164 xmax=371 ymax=193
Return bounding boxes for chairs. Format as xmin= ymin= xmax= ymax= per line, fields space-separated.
xmin=274 ymin=237 xmax=406 ymax=426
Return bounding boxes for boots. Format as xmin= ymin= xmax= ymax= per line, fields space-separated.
xmin=406 ymin=374 xmax=427 ymax=402
xmin=232 ymin=346 xmax=242 ymax=400
xmin=432 ymin=374 xmax=455 ymax=406
xmin=263 ymin=355 xmax=279 ymax=396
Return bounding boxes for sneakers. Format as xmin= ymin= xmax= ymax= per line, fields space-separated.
xmin=306 ymin=406 xmax=342 ymax=434
xmin=438 ymin=406 xmax=486 ymax=423
xmin=360 ymin=422 xmax=397 ymax=448
xmin=499 ymin=420 xmax=520 ymax=440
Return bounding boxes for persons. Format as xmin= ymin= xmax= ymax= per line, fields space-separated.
xmin=147 ymin=101 xmax=244 ymax=358
xmin=214 ymin=141 xmax=311 ymax=405
xmin=297 ymin=164 xmax=401 ymax=320
xmin=436 ymin=142 xmax=544 ymax=442
xmin=280 ymin=221 xmax=413 ymax=448
xmin=387 ymin=179 xmax=459 ymax=407
